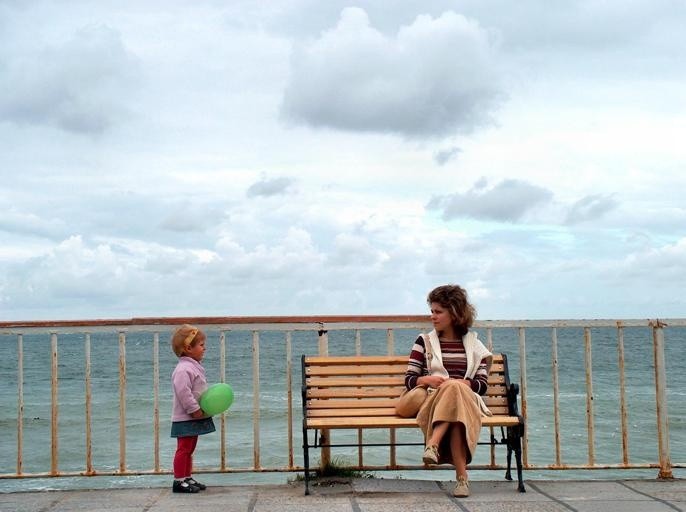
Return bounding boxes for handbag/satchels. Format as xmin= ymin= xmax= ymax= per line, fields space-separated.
xmin=396 ymin=335 xmax=433 ymax=418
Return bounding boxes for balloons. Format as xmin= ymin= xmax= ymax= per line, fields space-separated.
xmin=200 ymin=383 xmax=234 ymax=417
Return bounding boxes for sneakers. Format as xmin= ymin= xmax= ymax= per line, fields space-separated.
xmin=451 ymin=473 xmax=471 ymax=497
xmin=422 ymin=442 xmax=440 ymax=467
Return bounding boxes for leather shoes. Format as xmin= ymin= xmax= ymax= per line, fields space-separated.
xmin=172 ymin=479 xmax=200 ymax=493
xmin=186 ymin=477 xmax=207 ymax=490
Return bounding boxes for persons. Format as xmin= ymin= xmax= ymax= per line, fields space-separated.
xmin=404 ymin=285 xmax=494 ymax=499
xmin=169 ymin=325 xmax=217 ymax=493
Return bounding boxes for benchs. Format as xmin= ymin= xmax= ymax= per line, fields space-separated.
xmin=301 ymin=353 xmax=527 ymax=495
